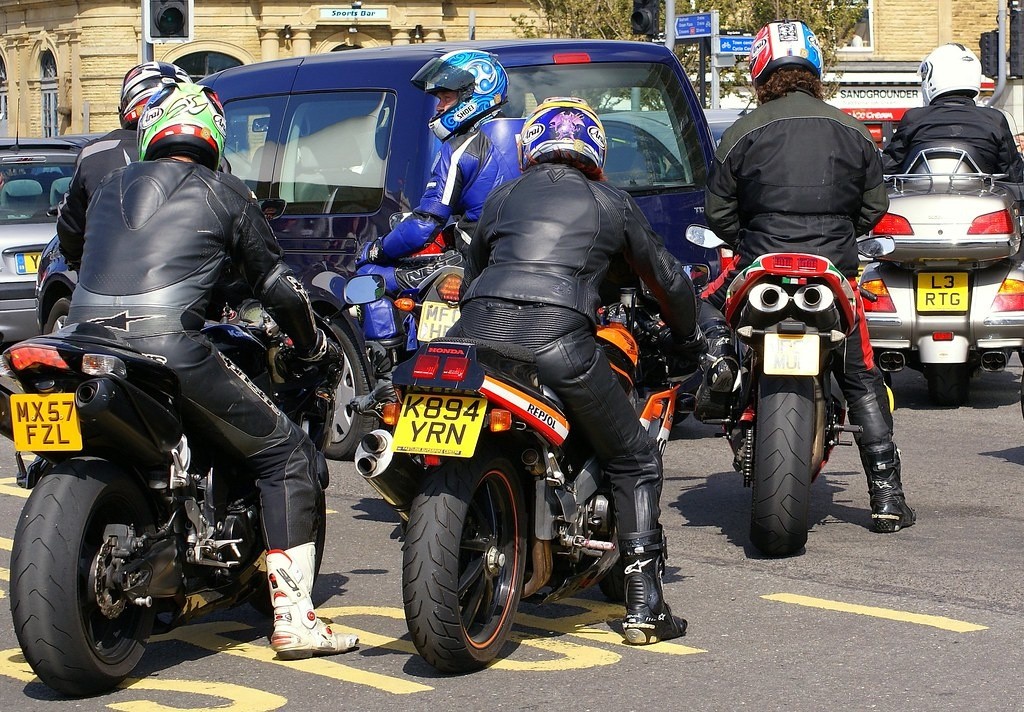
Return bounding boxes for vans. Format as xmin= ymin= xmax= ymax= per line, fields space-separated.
xmin=34 ymin=35 xmax=720 ymax=463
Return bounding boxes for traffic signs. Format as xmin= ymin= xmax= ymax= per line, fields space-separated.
xmin=674 ymin=13 xmax=711 ymax=39
xmin=719 ymin=36 xmax=756 ymax=56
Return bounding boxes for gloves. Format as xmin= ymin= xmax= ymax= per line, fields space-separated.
xmin=353 ymin=234 xmax=385 ymax=271
xmin=683 ymin=324 xmax=709 ymax=361
xmin=313 ymin=336 xmax=346 ymax=381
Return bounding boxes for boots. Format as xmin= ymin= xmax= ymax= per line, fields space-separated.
xmin=265 ymin=542 xmax=360 ymax=661
xmin=859 ymin=435 xmax=916 ymax=532
xmin=693 ymin=320 xmax=739 ymax=423
xmin=349 ymin=335 xmax=408 ymax=422
xmin=615 ymin=523 xmax=688 ymax=645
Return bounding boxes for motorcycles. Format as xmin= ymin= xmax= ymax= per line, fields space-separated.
xmin=353 ymin=210 xmax=704 ymax=426
xmin=1 ymin=272 xmax=391 ymax=698
xmin=858 ymin=138 xmax=1024 ymax=408
xmin=352 ymin=262 xmax=716 ymax=675
xmin=683 ymin=223 xmax=898 ymax=557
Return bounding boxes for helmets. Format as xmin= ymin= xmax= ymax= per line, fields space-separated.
xmin=515 ymin=95 xmax=607 ymax=173
xmin=748 ymin=19 xmax=825 ymax=89
xmin=117 ymin=61 xmax=194 ymax=129
xmin=409 ymin=48 xmax=511 ymax=142
xmin=137 ymin=83 xmax=227 ymax=173
xmin=915 ymin=43 xmax=983 ymax=104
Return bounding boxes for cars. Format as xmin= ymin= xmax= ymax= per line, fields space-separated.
xmin=0 ymin=134 xmax=83 ymax=345
xmin=598 ymin=106 xmax=752 ymax=185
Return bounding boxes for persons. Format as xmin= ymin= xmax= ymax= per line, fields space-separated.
xmin=848 ymin=28 xmax=863 ymax=47
xmin=55 ymin=82 xmax=360 ymax=661
xmin=880 ymin=42 xmax=1024 ymax=184
xmin=443 ymin=96 xmax=700 ymax=646
xmin=348 ymin=50 xmax=520 ymax=416
xmin=694 ymin=22 xmax=916 ymax=534
xmin=57 ymin=59 xmax=194 ymax=285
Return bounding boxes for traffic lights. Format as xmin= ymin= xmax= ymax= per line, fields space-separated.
xmin=139 ymin=0 xmax=194 ymax=45
xmin=630 ymin=0 xmax=659 ymax=37
